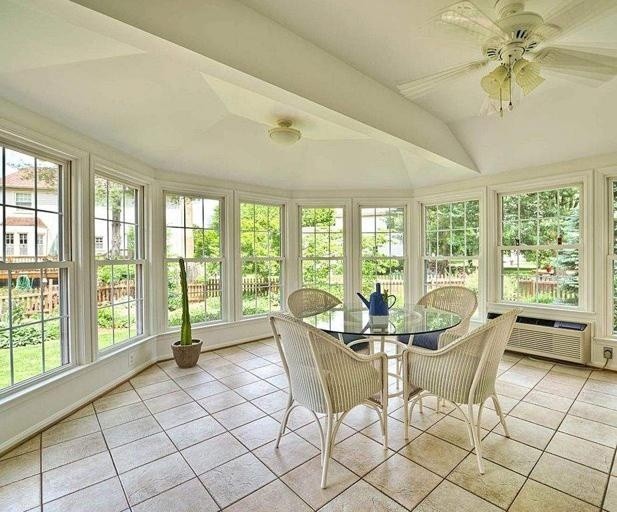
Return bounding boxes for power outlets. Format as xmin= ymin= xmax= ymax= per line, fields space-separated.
xmin=602 ymin=346 xmax=613 ymax=360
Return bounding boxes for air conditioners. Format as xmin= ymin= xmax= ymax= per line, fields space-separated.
xmin=486 ymin=313 xmax=591 ymax=365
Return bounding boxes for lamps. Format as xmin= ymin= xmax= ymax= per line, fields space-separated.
xmin=480 ymin=49 xmax=546 ymax=118
xmin=265 ymin=118 xmax=302 ymax=148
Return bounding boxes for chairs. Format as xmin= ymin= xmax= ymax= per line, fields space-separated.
xmin=266 ymin=310 xmax=390 ymax=490
xmin=396 ymin=285 xmax=478 ymax=413
xmin=288 ymin=287 xmax=371 ymax=357
xmin=401 ymin=306 xmax=520 ymax=476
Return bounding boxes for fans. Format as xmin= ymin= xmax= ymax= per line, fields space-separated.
xmin=395 ymin=1 xmax=617 ymax=101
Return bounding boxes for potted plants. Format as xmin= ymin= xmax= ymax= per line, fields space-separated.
xmin=170 ymin=257 xmax=203 ymax=369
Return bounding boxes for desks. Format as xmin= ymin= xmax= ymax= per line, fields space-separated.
xmin=282 ymin=301 xmax=464 ymax=423
